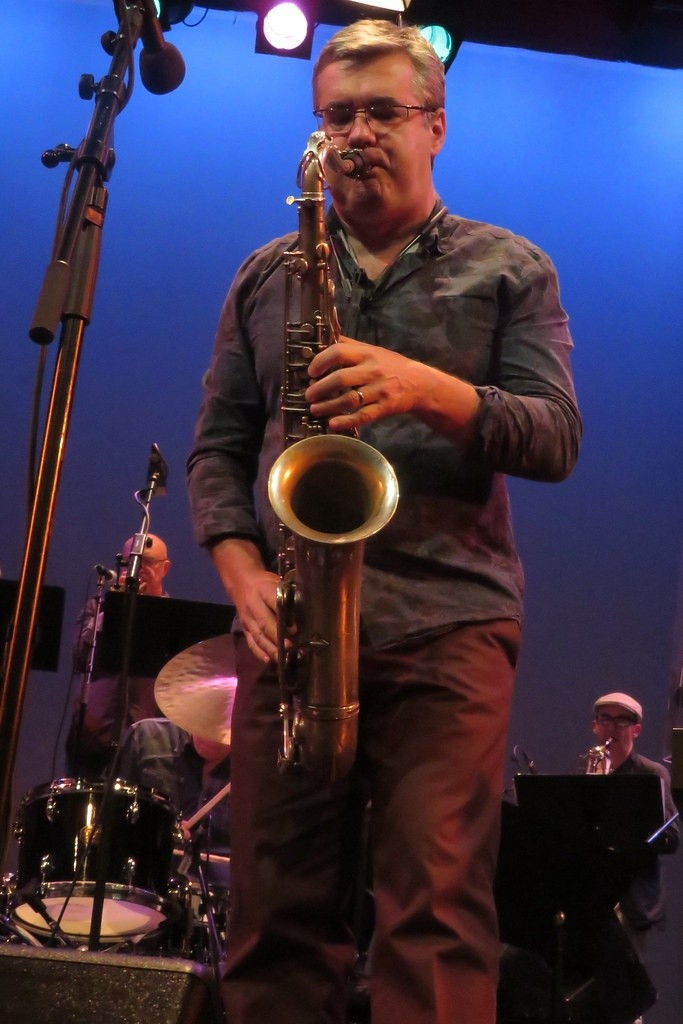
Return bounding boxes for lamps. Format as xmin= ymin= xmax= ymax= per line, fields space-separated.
xmin=403 ymin=0 xmax=471 ymax=76
xmin=254 ymin=0 xmax=319 ymax=61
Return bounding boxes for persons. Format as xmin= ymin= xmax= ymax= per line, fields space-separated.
xmin=65 ymin=532 xmax=187 ymax=896
xmin=582 ymin=690 xmax=682 ymax=1024
xmin=182 ymin=15 xmax=584 ymax=1023
xmin=171 ymin=733 xmax=231 ymax=881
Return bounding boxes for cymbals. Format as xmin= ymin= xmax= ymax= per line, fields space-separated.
xmin=152 ymin=631 xmax=237 ymax=749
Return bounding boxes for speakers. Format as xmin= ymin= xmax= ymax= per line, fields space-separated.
xmin=0 ymin=942 xmax=226 ymax=1024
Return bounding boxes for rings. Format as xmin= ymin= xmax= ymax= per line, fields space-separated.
xmin=355 ymin=389 xmax=365 ymax=407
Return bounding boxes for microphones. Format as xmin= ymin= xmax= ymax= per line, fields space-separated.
xmin=147 ymin=447 xmax=167 ymax=496
xmin=96 ymin=564 xmax=117 ymax=584
xmin=521 ymin=751 xmax=539 ymax=774
xmin=127 ymin=0 xmax=186 ymax=95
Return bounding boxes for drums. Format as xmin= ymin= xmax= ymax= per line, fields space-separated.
xmin=6 ymin=772 xmax=185 ymax=944
xmin=167 ymin=880 xmax=230 ymax=941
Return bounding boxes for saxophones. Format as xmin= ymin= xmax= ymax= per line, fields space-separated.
xmin=580 ymin=737 xmax=615 ymax=775
xmin=265 ymin=124 xmax=405 ymax=788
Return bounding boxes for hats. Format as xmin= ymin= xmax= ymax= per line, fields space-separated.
xmin=594 ymin=693 xmax=642 ymax=722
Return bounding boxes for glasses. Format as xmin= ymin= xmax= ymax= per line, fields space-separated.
xmin=143 ymin=559 xmax=164 ymax=568
xmin=596 ymin=713 xmax=636 ymax=727
xmin=313 ymin=104 xmax=436 ymax=133
